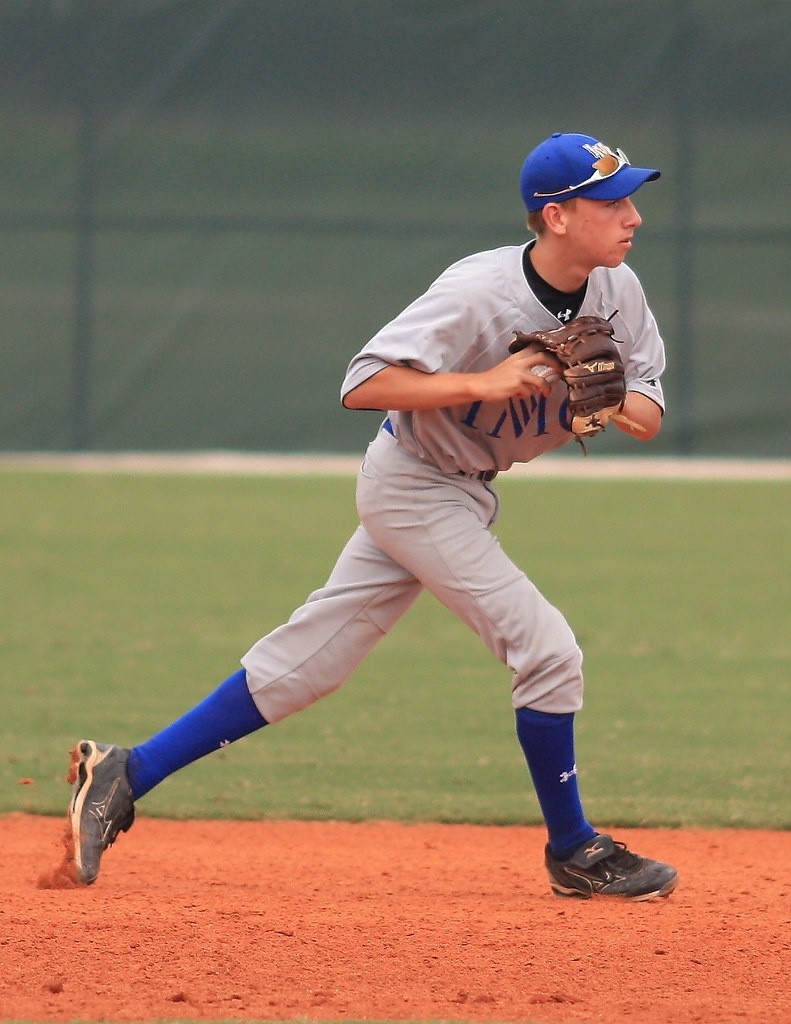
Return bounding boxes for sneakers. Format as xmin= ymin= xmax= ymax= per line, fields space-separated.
xmin=61 ymin=739 xmax=138 ymax=886
xmin=544 ymin=835 xmax=680 ymax=903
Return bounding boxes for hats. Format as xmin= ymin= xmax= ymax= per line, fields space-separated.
xmin=519 ymin=130 xmax=663 ymax=212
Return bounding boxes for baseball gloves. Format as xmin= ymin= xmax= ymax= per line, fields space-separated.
xmin=507 ymin=317 xmax=628 ymax=436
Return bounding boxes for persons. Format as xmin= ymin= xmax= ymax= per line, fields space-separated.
xmin=66 ymin=133 xmax=680 ymax=900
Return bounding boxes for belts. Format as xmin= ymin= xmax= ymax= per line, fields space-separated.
xmin=383 ymin=420 xmax=498 ymax=483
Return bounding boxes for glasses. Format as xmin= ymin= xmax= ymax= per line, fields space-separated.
xmin=534 ymin=147 xmax=631 ymax=199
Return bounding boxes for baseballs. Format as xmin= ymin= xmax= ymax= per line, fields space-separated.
xmin=529 ymin=365 xmax=560 ymax=387
